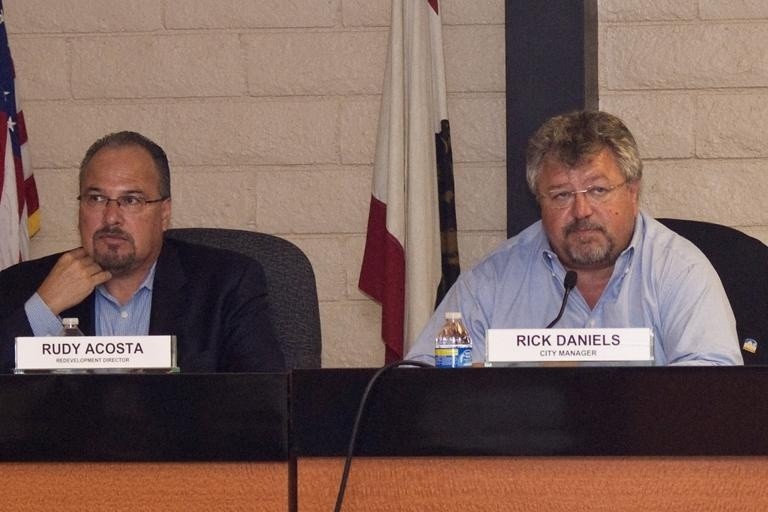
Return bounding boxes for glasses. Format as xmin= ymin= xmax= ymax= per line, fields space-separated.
xmin=535 ymin=179 xmax=628 ymax=209
xmin=77 ymin=194 xmax=165 ymax=214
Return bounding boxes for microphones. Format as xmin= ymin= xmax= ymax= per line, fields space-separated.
xmin=544 ymin=271 xmax=578 ymax=329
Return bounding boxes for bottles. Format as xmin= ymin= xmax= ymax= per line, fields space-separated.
xmin=434 ymin=312 xmax=472 ymax=367
xmin=54 ymin=318 xmax=87 ymax=374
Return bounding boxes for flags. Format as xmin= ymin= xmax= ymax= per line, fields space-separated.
xmin=357 ymin=1 xmax=462 ymax=368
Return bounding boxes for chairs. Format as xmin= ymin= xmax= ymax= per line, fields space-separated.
xmin=163 ymin=228 xmax=322 ymax=368
xmin=658 ymin=217 xmax=768 ymax=364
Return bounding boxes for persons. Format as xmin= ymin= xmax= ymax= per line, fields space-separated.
xmin=1 ymin=130 xmax=286 ymax=376
xmin=396 ymin=108 xmax=746 ymax=370
xmin=0 ymin=2 xmax=44 ymax=272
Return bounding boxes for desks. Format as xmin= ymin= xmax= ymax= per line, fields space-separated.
xmin=2 ymin=366 xmax=768 ymax=512
